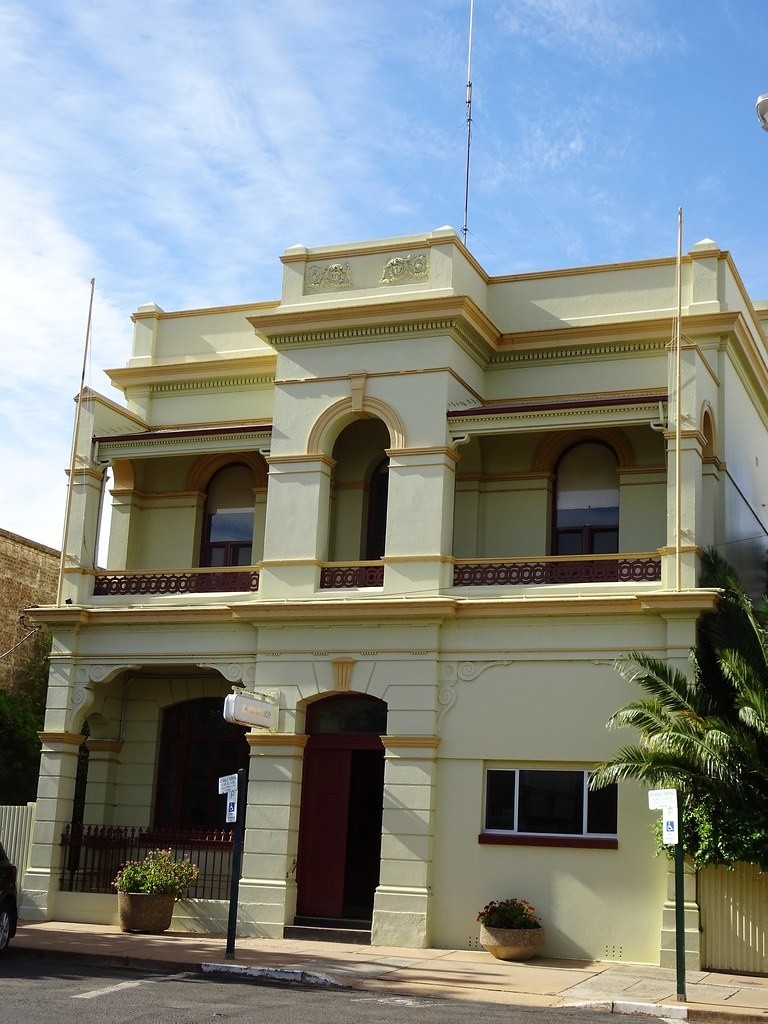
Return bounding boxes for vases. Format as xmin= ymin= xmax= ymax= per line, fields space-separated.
xmin=480 ymin=924 xmax=544 ymax=960
xmin=117 ymin=891 xmax=175 ymax=933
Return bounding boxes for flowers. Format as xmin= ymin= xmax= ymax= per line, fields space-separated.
xmin=111 ymin=847 xmax=199 ymax=901
xmin=476 ymin=898 xmax=537 ymax=927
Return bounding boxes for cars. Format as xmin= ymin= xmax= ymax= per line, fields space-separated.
xmin=0 ymin=843 xmax=19 ymax=955
